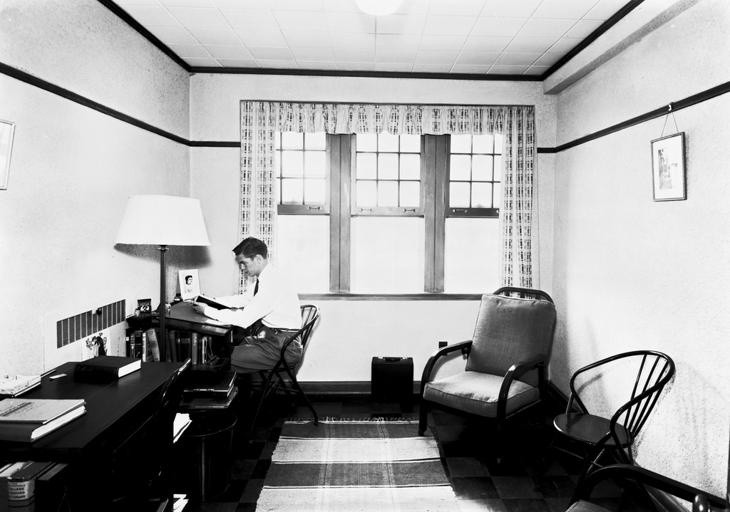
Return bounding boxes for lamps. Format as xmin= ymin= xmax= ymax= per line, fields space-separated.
xmin=116 ymin=193 xmax=211 ymax=356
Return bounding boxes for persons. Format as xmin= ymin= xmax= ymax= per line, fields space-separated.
xmin=185 ymin=274 xmax=195 ymax=298
xmin=194 ymin=236 xmax=304 ymax=381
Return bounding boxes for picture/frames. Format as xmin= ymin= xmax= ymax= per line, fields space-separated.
xmin=649 ymin=131 xmax=689 ymax=203
xmin=0 ymin=118 xmax=19 ymax=190
xmin=177 ymin=266 xmax=202 ymax=301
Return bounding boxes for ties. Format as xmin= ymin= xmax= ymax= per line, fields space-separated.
xmin=254 ymin=279 xmax=259 ymax=295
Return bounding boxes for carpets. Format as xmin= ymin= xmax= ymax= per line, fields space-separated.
xmin=252 ymin=413 xmax=570 ymax=512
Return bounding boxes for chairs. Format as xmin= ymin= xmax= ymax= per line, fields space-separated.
xmin=541 ymin=348 xmax=676 ymax=500
xmin=416 ymin=286 xmax=558 ymax=470
xmin=563 ymin=459 xmax=729 ymax=511
xmin=247 ymin=302 xmax=323 ymax=433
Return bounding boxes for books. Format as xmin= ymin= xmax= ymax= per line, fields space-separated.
xmin=76 ymin=356 xmax=143 ymax=380
xmin=0 ymin=396 xmax=89 ymax=442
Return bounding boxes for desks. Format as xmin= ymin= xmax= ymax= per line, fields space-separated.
xmin=0 ymin=355 xmax=198 ymax=512
xmin=127 ymin=294 xmax=248 ymax=487
